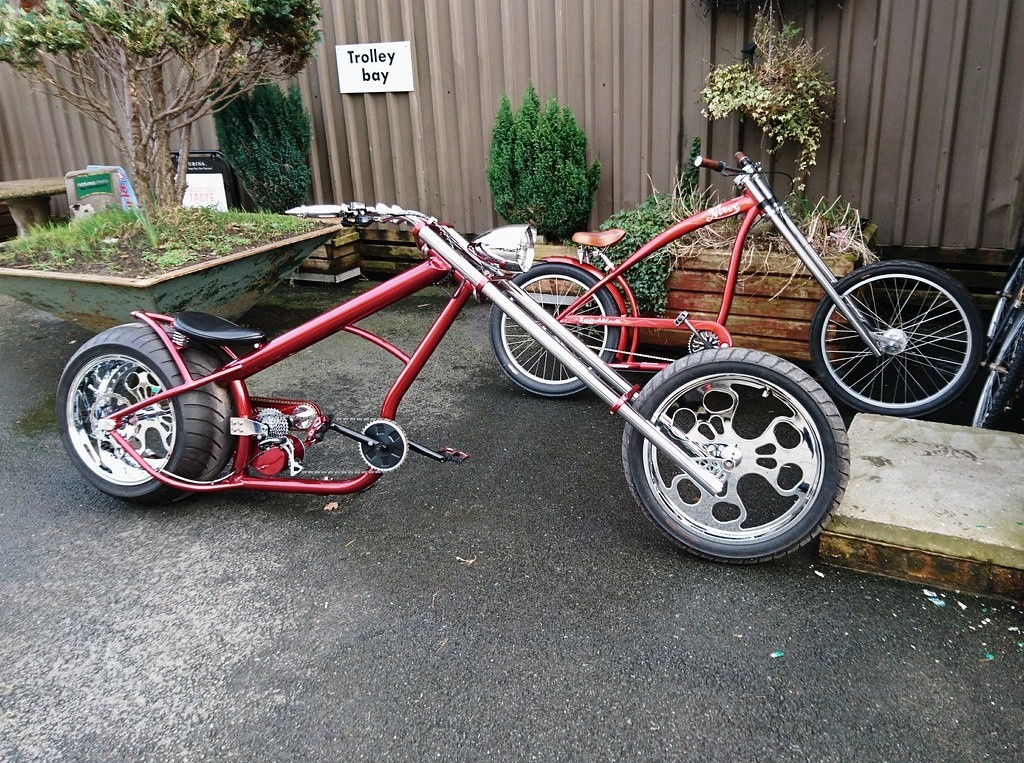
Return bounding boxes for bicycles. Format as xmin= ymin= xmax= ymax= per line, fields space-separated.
xmin=484 ymin=152 xmax=987 ymax=430
xmin=52 ymin=200 xmax=851 ymax=573
xmin=971 ymin=254 xmax=1024 ymax=431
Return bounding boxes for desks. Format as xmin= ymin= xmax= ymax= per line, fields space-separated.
xmin=0 ymin=177 xmax=67 ymax=238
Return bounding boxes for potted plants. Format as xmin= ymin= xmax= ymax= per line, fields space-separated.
xmin=0 ymin=0 xmax=346 ymax=334
xmin=210 ymin=73 xmax=363 ymax=284
xmin=591 ymin=136 xmax=880 ymax=366
xmin=693 ymin=0 xmax=840 ymax=202
xmin=487 ymin=80 xmax=604 ymax=304
